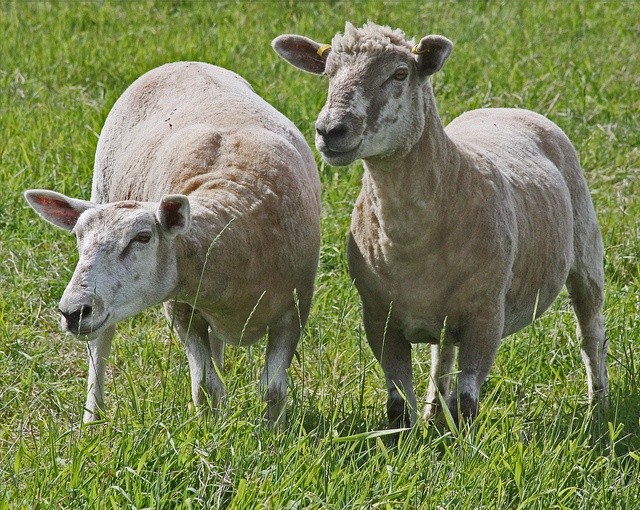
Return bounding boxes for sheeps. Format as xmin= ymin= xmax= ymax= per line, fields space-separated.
xmin=269 ymin=17 xmax=610 ymax=446
xmin=23 ymin=60 xmax=323 ymax=434
